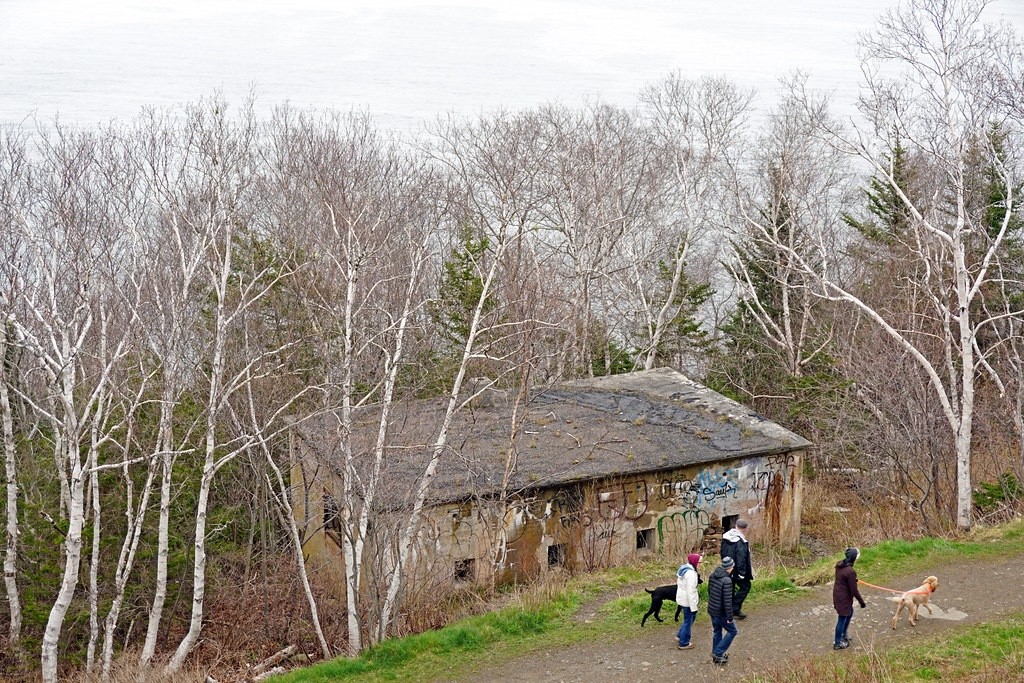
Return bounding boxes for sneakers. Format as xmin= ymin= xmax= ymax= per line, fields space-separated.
xmin=845 ymin=635 xmax=852 ymax=641
xmin=833 ymin=641 xmax=849 ymax=650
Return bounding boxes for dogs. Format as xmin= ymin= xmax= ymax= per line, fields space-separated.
xmin=884 ymin=575 xmax=939 ymax=631
xmin=642 ymin=574 xmax=704 ymax=628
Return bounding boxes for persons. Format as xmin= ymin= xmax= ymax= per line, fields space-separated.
xmin=720 ymin=519 xmax=753 ymax=619
xmin=707 ymin=556 xmax=738 ymax=663
xmin=833 ymin=547 xmax=866 ymax=650
xmin=676 ymin=554 xmax=702 ymax=649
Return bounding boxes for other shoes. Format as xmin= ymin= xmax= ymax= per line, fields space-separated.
xmin=733 ymin=610 xmax=747 ymax=620
xmin=678 ymin=642 xmax=696 ymax=650
xmin=674 ymin=636 xmax=680 ymax=641
xmin=711 ymin=652 xmax=727 ymax=663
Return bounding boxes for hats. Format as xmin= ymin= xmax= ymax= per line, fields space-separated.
xmin=736 ymin=518 xmax=748 ymax=529
xmin=721 ymin=557 xmax=735 ymax=569
xmin=687 ymin=554 xmax=700 ymax=567
xmin=845 ymin=549 xmax=859 ymax=557
